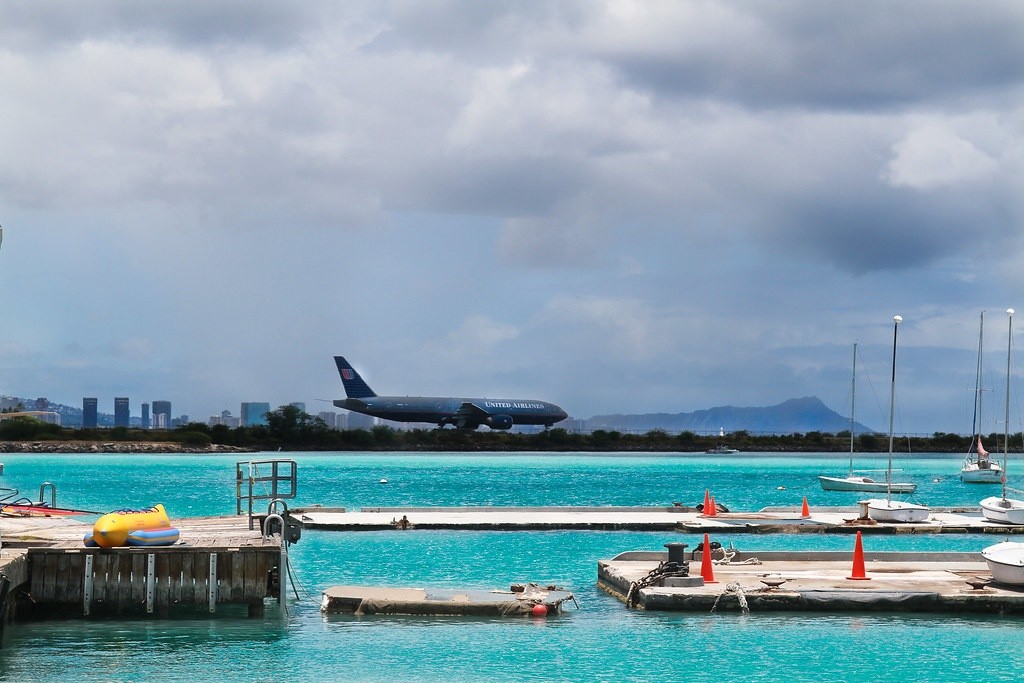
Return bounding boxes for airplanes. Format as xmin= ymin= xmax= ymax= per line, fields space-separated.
xmin=333 ymin=355 xmax=568 ymax=430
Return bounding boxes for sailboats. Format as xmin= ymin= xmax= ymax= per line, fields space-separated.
xmin=818 ymin=342 xmax=918 ymax=492
xmin=960 ymin=311 xmax=1004 ymax=483
xmin=979 ymin=308 xmax=1024 ymax=525
xmin=867 ymin=315 xmax=931 ymax=521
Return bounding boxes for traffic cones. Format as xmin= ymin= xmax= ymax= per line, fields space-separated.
xmin=707 ymin=497 xmax=719 ymax=518
xmin=701 ymin=532 xmax=721 ymax=584
xmin=846 ymin=531 xmax=870 ymax=580
xmin=701 ymin=488 xmax=710 ymax=516
xmin=800 ymin=496 xmax=811 ymax=518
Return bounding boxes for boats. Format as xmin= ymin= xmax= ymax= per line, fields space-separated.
xmin=981 ymin=541 xmax=1024 ymax=585
xmin=83 ymin=504 xmax=180 ymax=547
